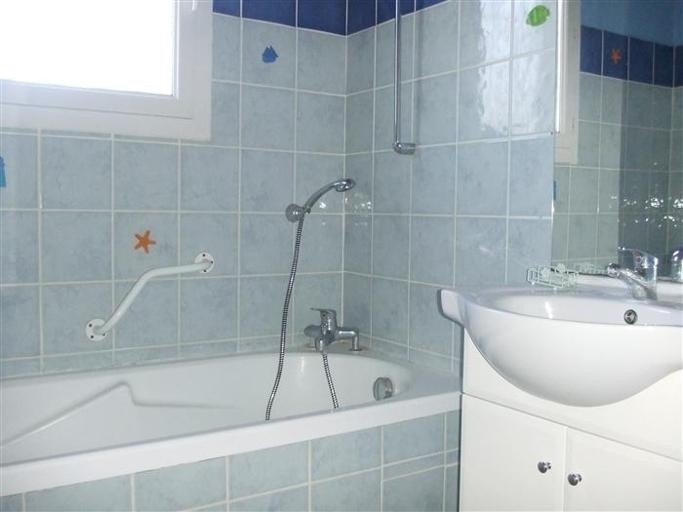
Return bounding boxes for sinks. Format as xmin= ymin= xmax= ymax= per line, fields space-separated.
xmin=440 ymin=281 xmax=682 ymax=408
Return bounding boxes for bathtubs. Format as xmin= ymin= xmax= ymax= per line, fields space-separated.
xmin=0 ymin=347 xmax=460 ymax=493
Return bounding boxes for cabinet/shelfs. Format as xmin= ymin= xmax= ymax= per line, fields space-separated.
xmin=457 ymin=393 xmax=682 ymax=512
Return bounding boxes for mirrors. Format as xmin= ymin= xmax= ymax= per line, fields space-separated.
xmin=546 ymin=0 xmax=682 ymax=282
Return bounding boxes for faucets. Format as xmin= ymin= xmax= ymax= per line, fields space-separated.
xmin=311 ymin=306 xmax=336 ymax=353
xmin=605 ymin=247 xmax=658 ymax=300
xmin=670 ymin=251 xmax=679 ymax=278
